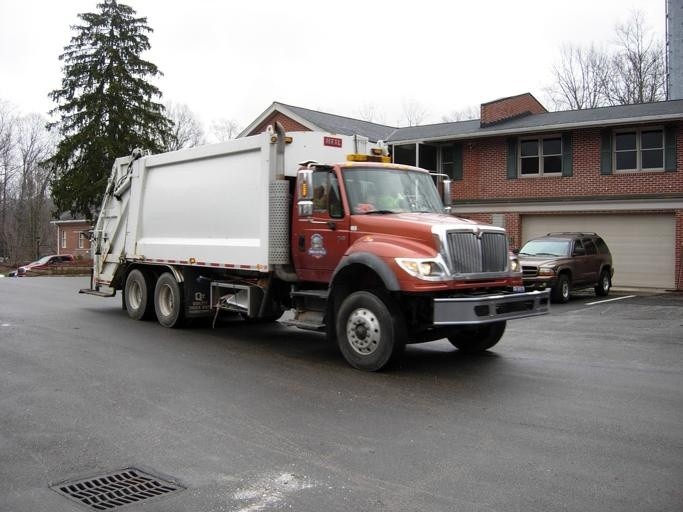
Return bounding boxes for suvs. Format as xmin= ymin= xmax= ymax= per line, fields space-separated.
xmin=518 ymin=231 xmax=614 ymax=304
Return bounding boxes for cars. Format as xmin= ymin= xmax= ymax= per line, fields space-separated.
xmin=8 ymin=253 xmax=75 ymax=276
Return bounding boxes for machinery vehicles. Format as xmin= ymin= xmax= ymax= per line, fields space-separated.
xmin=78 ymin=118 xmax=552 ymax=373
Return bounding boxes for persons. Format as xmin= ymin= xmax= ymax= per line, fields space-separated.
xmin=376 ymin=181 xmax=408 ymax=209
xmin=312 ymin=181 xmax=339 ymax=214
